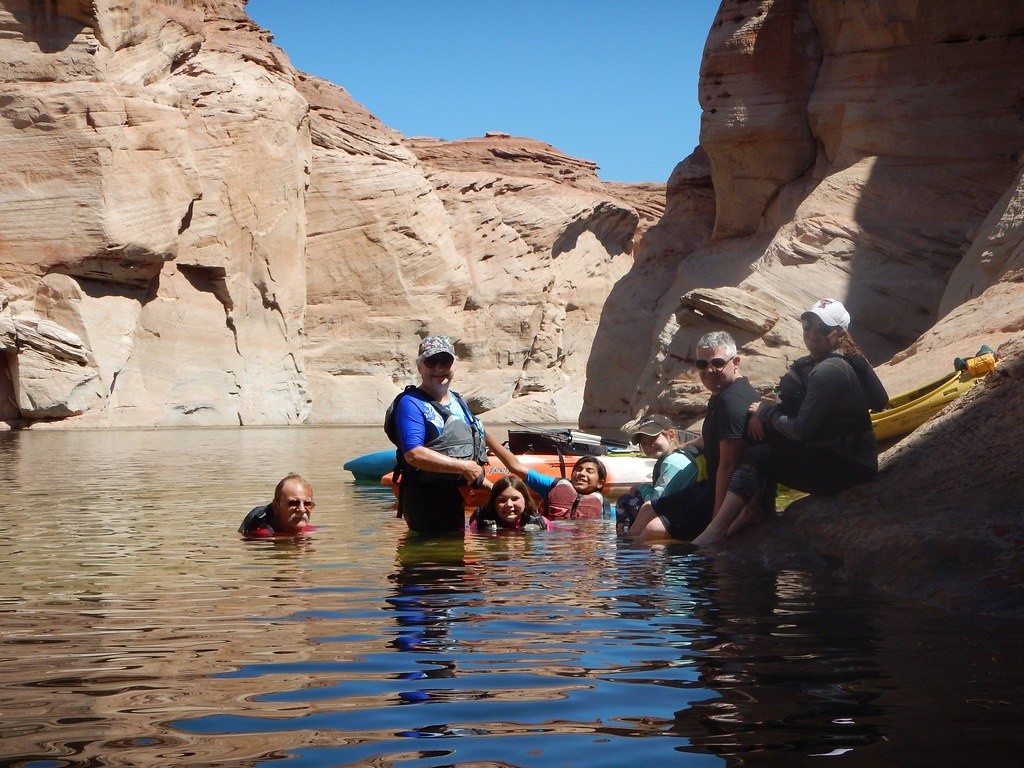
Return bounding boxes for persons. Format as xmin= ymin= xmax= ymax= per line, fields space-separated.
xmin=393 ymin=335 xmax=494 ymax=535
xmin=469 ymin=475 xmax=555 ymax=530
xmin=617 ymin=414 xmax=713 ymax=538
xmin=483 ymin=426 xmax=615 ymax=519
xmin=678 ymin=331 xmax=779 ymax=520
xmin=692 ymin=298 xmax=878 ymax=544
xmin=249 ymin=474 xmax=315 ymax=535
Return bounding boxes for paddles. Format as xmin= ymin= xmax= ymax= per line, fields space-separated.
xmin=509 ymin=418 xmax=640 ymax=450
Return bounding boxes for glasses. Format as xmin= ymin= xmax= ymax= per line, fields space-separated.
xmin=288 ymin=499 xmax=316 ymax=510
xmin=424 ymin=356 xmax=451 ymax=368
xmin=802 ymin=319 xmax=832 ymax=335
xmin=695 ymin=357 xmax=735 ymax=370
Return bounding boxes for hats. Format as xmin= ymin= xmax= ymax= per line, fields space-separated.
xmin=631 ymin=414 xmax=672 ymax=445
xmin=418 ymin=335 xmax=455 ymax=359
xmin=800 ymin=299 xmax=850 ymax=332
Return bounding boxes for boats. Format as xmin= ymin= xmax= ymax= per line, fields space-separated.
xmin=867 ymin=356 xmax=998 ymax=442
xmin=343 ymin=345 xmax=801 ymax=514
